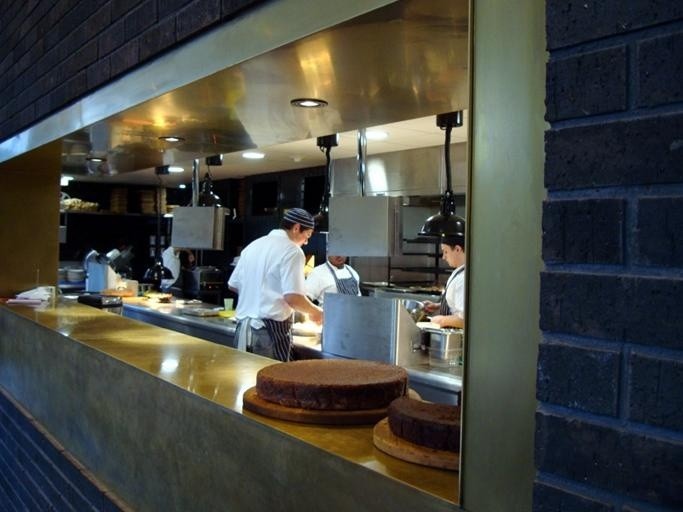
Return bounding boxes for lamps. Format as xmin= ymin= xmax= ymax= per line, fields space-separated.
xmin=311 ymin=133 xmax=339 ymax=234
xmin=417 ymin=111 xmax=464 ymax=238
xmin=187 ymin=154 xmax=224 ymax=209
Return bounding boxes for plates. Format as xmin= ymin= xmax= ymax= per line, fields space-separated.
xmin=67 ymin=269 xmax=84 ymax=282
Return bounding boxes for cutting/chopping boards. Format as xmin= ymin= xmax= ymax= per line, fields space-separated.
xmin=242 ymin=386 xmax=459 ymax=480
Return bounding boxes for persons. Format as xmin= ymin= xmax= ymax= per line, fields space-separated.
xmin=160 ymin=246 xmax=195 ymax=298
xmin=228 ymin=208 xmax=362 ymax=363
xmin=422 ymin=234 xmax=465 ymax=331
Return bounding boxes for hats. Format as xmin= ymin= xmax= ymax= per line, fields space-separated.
xmin=281 ymin=207 xmax=316 ymax=230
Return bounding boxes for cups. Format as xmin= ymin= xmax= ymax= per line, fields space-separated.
xmin=224 ymin=298 xmax=233 ymax=311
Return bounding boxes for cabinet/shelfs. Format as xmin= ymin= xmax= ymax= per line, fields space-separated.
xmin=387 ymin=199 xmax=453 ymax=289
